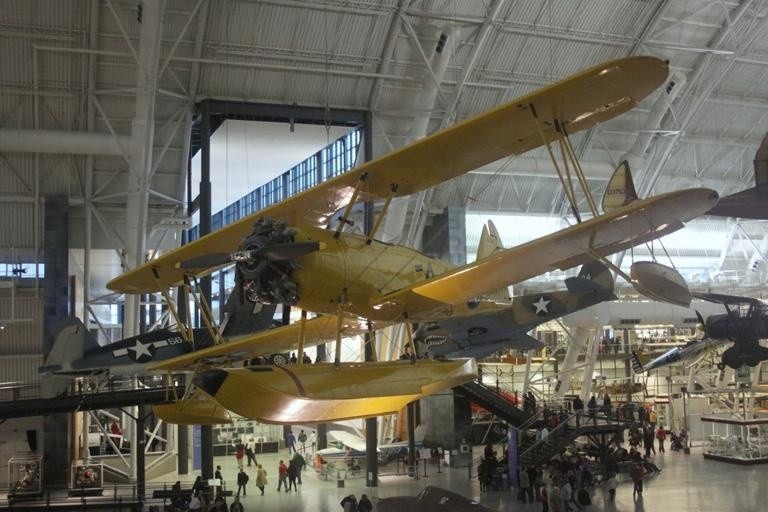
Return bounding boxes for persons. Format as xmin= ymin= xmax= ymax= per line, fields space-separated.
xmin=340 ymin=494 xmax=372 ymax=512
xmin=112 ymin=420 xmax=123 ymax=455
xmin=477 ymin=387 xmax=685 ymax=510
xmin=310 ymin=431 xmax=316 ymax=452
xmin=132 ymin=430 xmax=306 ymax=512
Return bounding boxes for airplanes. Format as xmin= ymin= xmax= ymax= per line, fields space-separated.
xmin=369 ymin=483 xmax=496 ymax=512
xmin=311 ymin=421 xmax=433 ymax=471
xmin=537 ymin=136 xmax=768 ymax=377
xmin=95 ymin=49 xmax=721 ymax=434
